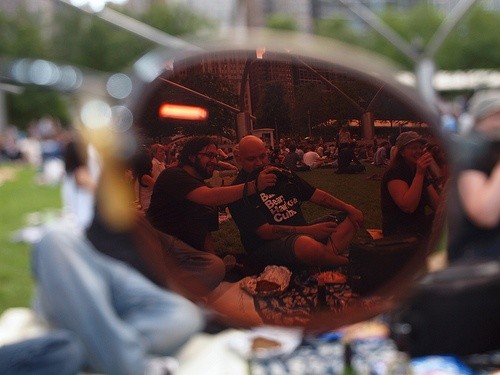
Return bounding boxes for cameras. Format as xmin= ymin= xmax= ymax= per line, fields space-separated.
xmin=272 ymin=169 xmax=296 ymax=184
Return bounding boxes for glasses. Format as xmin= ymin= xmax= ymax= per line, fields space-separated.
xmin=196 ymin=151 xmax=219 ymax=159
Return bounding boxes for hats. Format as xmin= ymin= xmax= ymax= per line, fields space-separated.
xmin=396 ymin=131 xmax=427 ymax=148
xmin=472 ymin=91 xmax=500 ymax=120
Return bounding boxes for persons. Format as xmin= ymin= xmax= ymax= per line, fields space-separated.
xmin=129 ymin=122 xmax=447 ymax=302
xmin=336 ymin=89 xmax=500 ymax=375
xmin=0 ymin=106 xmax=203 ymax=375
